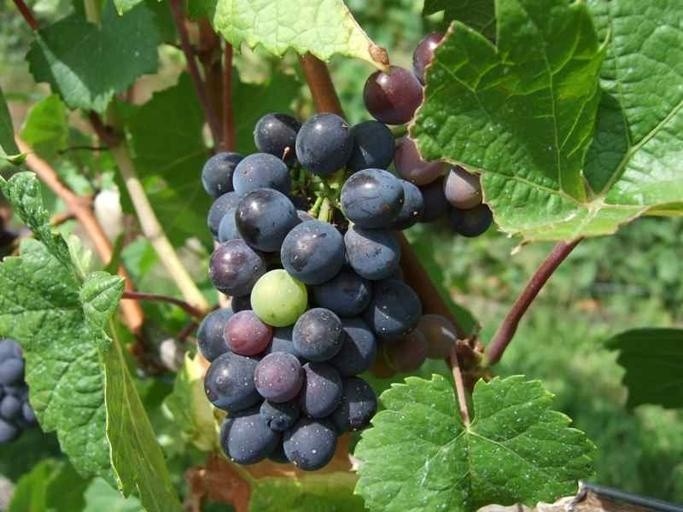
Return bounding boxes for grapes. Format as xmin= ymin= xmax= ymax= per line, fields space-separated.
xmin=0 ymin=336 xmax=39 ymax=446
xmin=198 ymin=31 xmax=496 ymax=473
